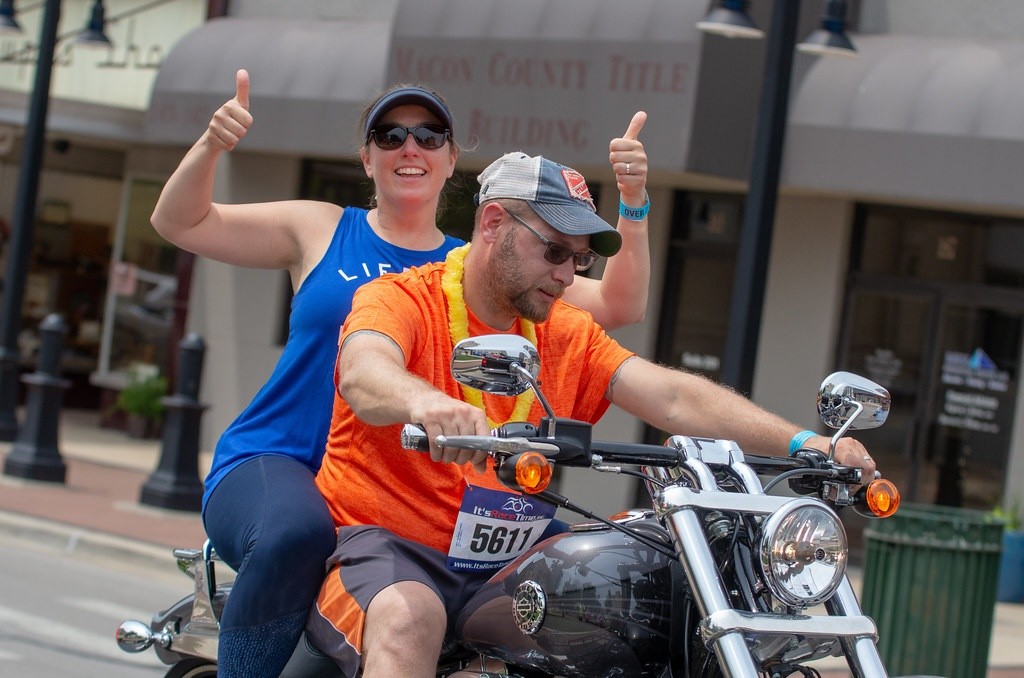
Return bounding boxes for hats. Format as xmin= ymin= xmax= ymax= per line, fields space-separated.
xmin=474 ymin=152 xmax=621 ymax=258
xmin=367 ymin=87 xmax=453 ymax=141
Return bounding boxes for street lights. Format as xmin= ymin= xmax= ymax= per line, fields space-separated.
xmin=1 ymin=1 xmax=120 ymax=440
xmin=694 ymin=1 xmax=864 ymax=404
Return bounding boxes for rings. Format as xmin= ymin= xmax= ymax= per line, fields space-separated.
xmin=864 ymin=457 xmax=871 ymax=461
xmin=626 ymin=162 xmax=629 ymax=174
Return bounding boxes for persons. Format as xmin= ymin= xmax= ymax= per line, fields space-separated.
xmin=821 ymin=392 xmax=851 ymax=416
xmin=872 ymin=401 xmax=887 ymax=422
xmin=304 ymin=150 xmax=876 ymax=678
xmin=151 ymin=68 xmax=649 ymax=678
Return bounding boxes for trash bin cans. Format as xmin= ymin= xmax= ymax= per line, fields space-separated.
xmin=862 ymin=502 xmax=1006 ymax=678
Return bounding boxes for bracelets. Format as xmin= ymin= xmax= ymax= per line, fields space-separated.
xmin=619 ymin=188 xmax=649 ymax=223
xmin=789 ymin=430 xmax=817 ymax=454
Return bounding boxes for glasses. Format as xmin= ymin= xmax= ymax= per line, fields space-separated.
xmin=367 ymin=123 xmax=451 ymax=150
xmin=504 ymin=207 xmax=600 ymax=272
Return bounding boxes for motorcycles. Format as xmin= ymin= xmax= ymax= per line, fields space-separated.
xmin=114 ymin=335 xmax=901 ymax=678
